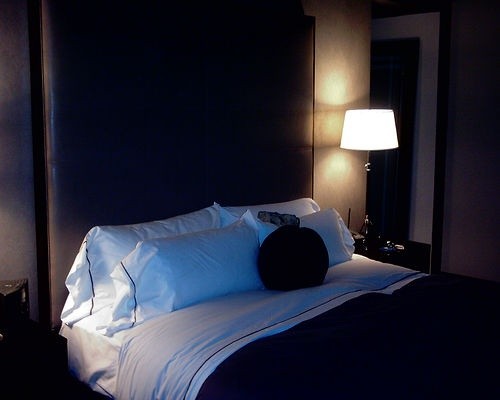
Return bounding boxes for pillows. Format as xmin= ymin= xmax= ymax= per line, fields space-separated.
xmin=216 ymin=196 xmax=321 ymax=218
xmin=104 ymin=208 xmax=265 ymax=337
xmin=256 ymin=207 xmax=356 ymax=268
xmin=60 ymin=201 xmax=240 ymax=326
xmin=254 ymin=224 xmax=330 ymax=291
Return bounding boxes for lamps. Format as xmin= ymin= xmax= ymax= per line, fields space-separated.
xmin=341 ymin=107 xmax=399 ymax=174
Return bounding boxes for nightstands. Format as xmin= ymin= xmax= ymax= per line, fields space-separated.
xmin=1 ymin=278 xmax=30 ymax=386
xmin=352 ymin=238 xmax=430 ymax=274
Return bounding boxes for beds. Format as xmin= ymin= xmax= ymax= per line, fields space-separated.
xmin=56 ymin=197 xmax=500 ymax=399
